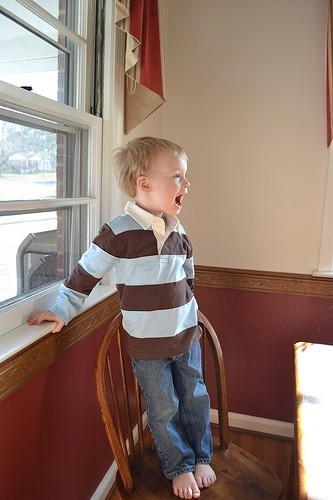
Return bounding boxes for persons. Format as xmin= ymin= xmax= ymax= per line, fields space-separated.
xmin=27 ymin=136 xmax=217 ymax=499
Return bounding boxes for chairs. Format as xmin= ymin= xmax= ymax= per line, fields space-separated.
xmin=95 ymin=310 xmax=285 ymax=500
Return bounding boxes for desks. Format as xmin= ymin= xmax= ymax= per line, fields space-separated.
xmin=292 ymin=340 xmax=333 ymax=500
